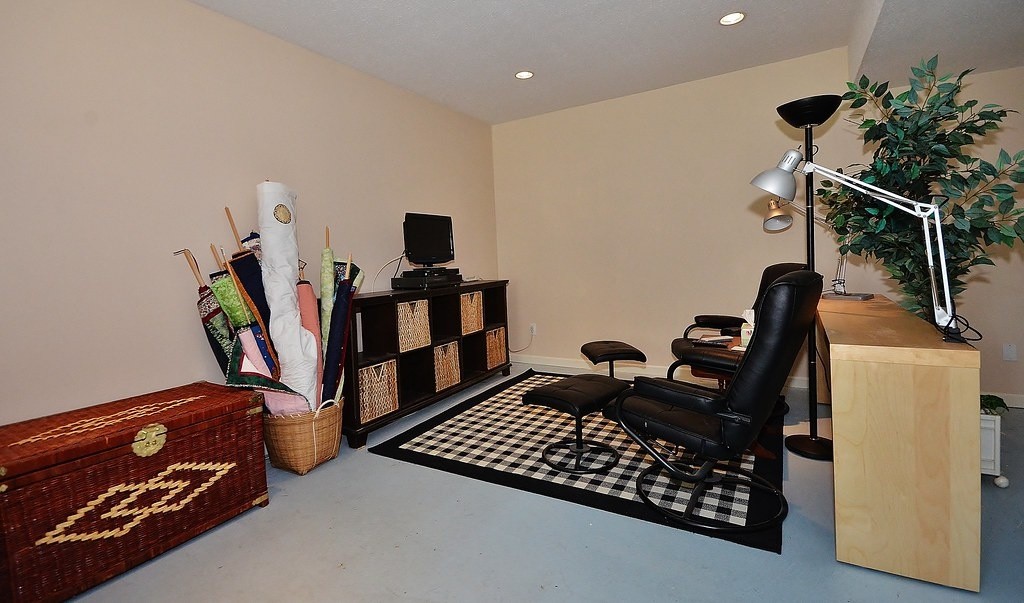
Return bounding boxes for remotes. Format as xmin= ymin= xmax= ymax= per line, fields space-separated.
xmin=701 ymin=335 xmax=733 ymax=342
xmin=692 ymin=340 xmax=729 ymax=348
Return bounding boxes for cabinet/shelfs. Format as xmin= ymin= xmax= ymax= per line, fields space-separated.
xmin=342 ymin=276 xmax=512 ymax=431
xmin=805 ymin=288 xmax=982 ymax=595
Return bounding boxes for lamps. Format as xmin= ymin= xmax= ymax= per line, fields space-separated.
xmin=748 ymin=145 xmax=985 ymax=343
xmin=777 ymin=94 xmax=842 ymax=462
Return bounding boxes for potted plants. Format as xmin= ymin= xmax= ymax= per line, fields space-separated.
xmin=823 ymin=53 xmax=1024 ymax=483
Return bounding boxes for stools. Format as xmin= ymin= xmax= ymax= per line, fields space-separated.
xmin=580 ymin=340 xmax=647 ymax=411
xmin=521 ymin=372 xmax=630 ymax=474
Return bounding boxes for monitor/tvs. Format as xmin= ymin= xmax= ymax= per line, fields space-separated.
xmin=401 ymin=212 xmax=455 ymax=270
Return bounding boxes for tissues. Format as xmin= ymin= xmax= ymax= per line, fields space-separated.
xmin=741 ymin=309 xmax=756 ymax=347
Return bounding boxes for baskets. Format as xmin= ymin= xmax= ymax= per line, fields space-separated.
xmin=261 ymin=392 xmax=345 ymax=475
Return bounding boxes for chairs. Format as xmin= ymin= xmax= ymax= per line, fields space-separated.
xmin=667 ymin=263 xmax=813 ymax=393
xmin=603 ymin=269 xmax=823 ymax=530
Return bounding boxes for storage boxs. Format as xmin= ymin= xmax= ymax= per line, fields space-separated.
xmin=0 ymin=378 xmax=269 ymax=603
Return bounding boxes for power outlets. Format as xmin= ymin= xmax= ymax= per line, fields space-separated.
xmin=529 ymin=322 xmax=536 ymax=336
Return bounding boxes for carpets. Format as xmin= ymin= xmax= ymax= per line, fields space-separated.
xmin=366 ymin=366 xmax=790 ymax=555
